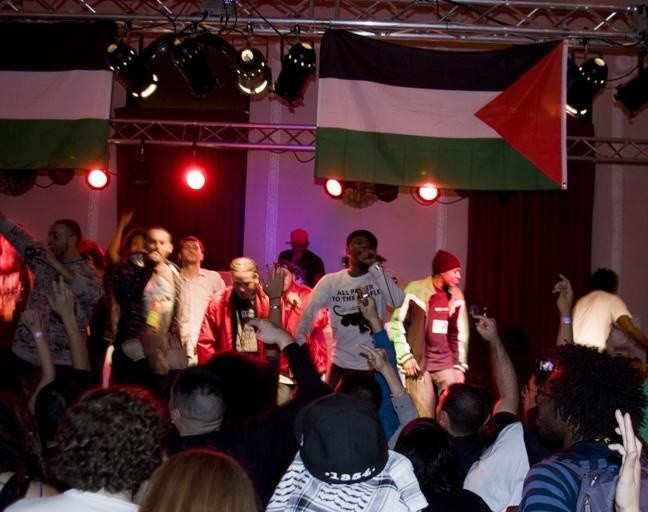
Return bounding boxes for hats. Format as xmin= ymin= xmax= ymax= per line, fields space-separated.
xmin=283 ymin=227 xmax=312 ymax=248
xmin=429 ymin=249 xmax=463 ymax=272
xmin=122 ymin=223 xmax=148 ymax=250
xmin=296 ymin=392 xmax=391 ymax=486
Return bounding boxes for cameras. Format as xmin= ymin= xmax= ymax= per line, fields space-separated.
xmin=535 ymin=358 xmax=558 ymax=375
xmin=355 ymin=289 xmax=369 ymax=306
xmin=24 ymin=247 xmax=41 ymax=256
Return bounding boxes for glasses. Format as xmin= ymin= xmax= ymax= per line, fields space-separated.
xmin=534 ymin=383 xmax=556 ymax=405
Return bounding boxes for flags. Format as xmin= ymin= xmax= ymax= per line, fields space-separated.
xmin=313 ymin=27 xmax=567 ymax=193
xmin=0 ymin=16 xmax=118 ymax=170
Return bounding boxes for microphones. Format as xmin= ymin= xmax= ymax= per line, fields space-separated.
xmin=368 ymin=253 xmax=386 ymax=262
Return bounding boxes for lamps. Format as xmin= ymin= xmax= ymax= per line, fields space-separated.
xmin=126 ymin=140 xmax=159 ymax=196
xmin=563 ymin=39 xmax=648 ymax=122
xmin=105 ymin=12 xmax=316 ymax=105
xmin=0 ymin=165 xmax=76 ymax=201
xmin=326 ymin=177 xmax=400 ymax=205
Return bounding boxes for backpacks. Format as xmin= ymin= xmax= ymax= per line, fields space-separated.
xmin=553 ymin=456 xmax=647 ymax=512
